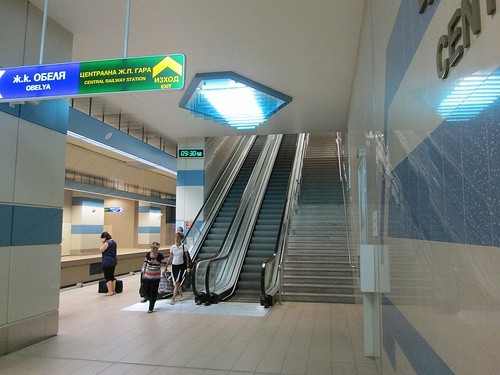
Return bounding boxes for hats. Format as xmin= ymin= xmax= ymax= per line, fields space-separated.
xmin=150 ymin=242 xmax=160 ymax=248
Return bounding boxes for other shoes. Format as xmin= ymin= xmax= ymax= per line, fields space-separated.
xmin=148 ymin=310 xmax=152 ymax=313
xmin=106 ymin=291 xmax=116 ymax=296
xmin=180 ymin=296 xmax=185 ymax=301
xmin=171 ymin=301 xmax=175 ymax=305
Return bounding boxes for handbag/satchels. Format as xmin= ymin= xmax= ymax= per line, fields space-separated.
xmin=163 ymin=266 xmax=171 ymax=279
xmin=139 ymin=285 xmax=144 ymax=297
xmin=183 ymin=243 xmax=193 ymax=269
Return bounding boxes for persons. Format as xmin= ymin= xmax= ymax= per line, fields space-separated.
xmin=100 ymin=231 xmax=117 ymax=296
xmin=141 ymin=241 xmax=167 ymax=312
xmin=177 ymin=227 xmax=187 ymax=244
xmin=163 ymin=232 xmax=191 ymax=304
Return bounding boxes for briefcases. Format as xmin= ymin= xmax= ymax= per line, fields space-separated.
xmin=98 ymin=279 xmax=123 ymax=293
